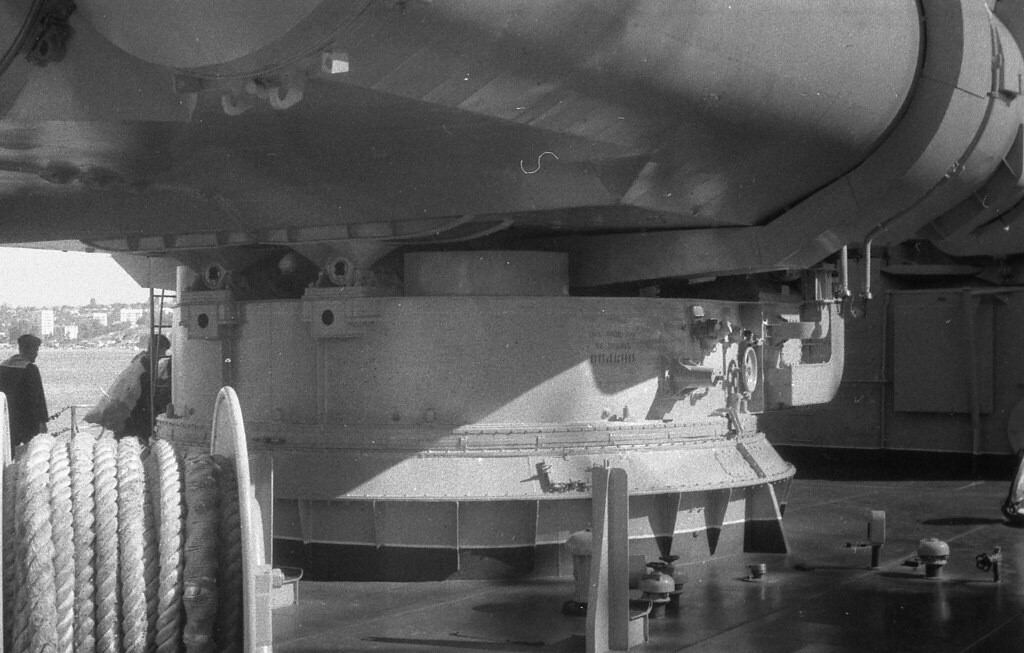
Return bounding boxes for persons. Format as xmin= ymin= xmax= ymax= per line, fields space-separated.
xmin=123 ymin=334 xmax=171 ymax=428
xmin=0 ymin=333 xmax=49 ymax=462
